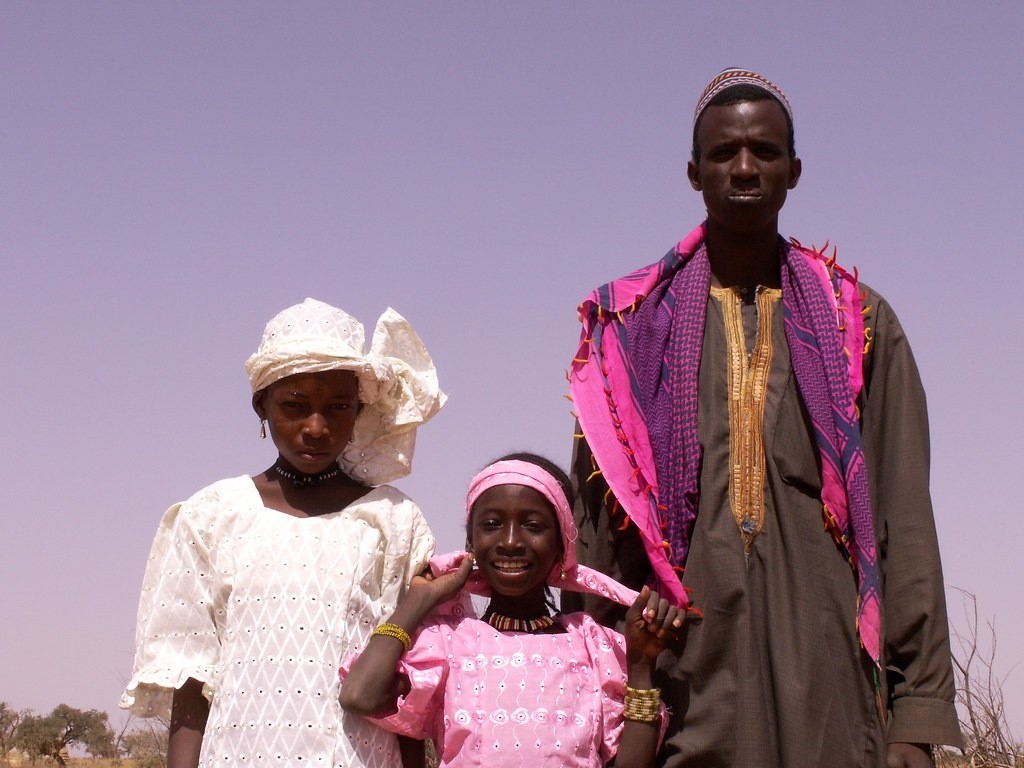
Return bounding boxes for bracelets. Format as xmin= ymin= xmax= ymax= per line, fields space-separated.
xmin=368 ymin=624 xmax=411 ymax=651
xmin=621 ymin=681 xmax=664 ymax=722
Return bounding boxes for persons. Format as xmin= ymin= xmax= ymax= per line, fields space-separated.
xmin=572 ymin=65 xmax=965 ymax=768
xmin=335 ymin=453 xmax=701 ymax=768
xmin=120 ymin=300 xmax=449 ymax=768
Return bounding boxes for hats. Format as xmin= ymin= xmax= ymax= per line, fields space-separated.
xmin=693 ymin=67 xmax=798 ymax=140
xmin=246 ymin=298 xmax=372 ymax=391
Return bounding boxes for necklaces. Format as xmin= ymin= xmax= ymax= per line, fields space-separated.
xmin=275 ymin=456 xmax=339 ymax=488
xmin=483 ymin=608 xmax=557 ymax=632
